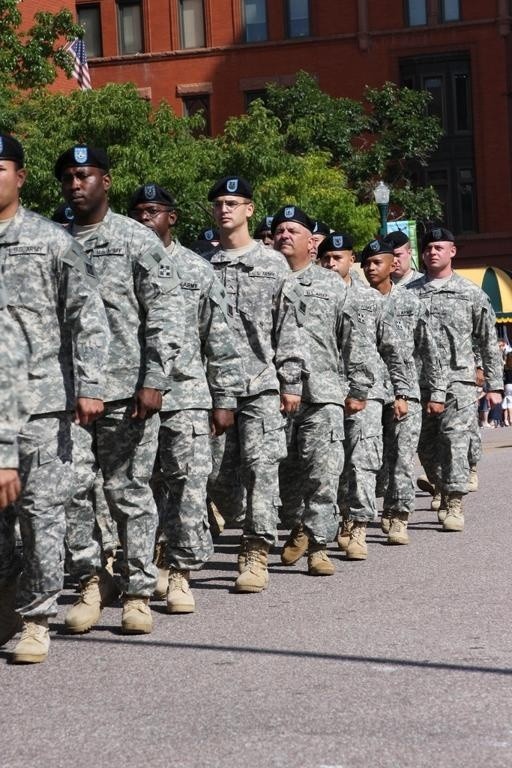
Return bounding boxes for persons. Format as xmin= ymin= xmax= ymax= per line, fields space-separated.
xmin=1 ymin=132 xmax=111 ymax=660
xmin=52 ymin=142 xmax=186 ymax=633
xmin=197 ymin=175 xmax=512 ymax=594
xmin=127 ymin=182 xmax=243 ymax=615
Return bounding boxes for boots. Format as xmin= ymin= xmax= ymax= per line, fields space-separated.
xmin=346 ymin=521 xmax=368 ymax=560
xmin=417 ymin=475 xmax=436 ymax=495
xmin=13 ymin=615 xmax=50 ymax=663
xmin=153 ymin=542 xmax=171 ymax=600
xmin=388 ymin=511 xmax=409 ymax=544
xmin=381 ymin=508 xmax=395 ymax=533
xmin=431 ymin=489 xmax=441 ymax=511
xmin=235 ymin=526 xmax=248 ymax=573
xmin=308 ymin=539 xmax=334 ymax=576
xmin=438 ymin=491 xmax=450 ymax=522
xmin=281 ymin=523 xmax=310 ymax=566
xmin=208 ymin=501 xmax=225 ymax=531
xmin=120 ymin=596 xmax=153 ymax=633
xmin=235 ymin=539 xmax=270 ymax=592
xmin=443 ymin=494 xmax=465 ymax=531
xmin=0 ymin=583 xmax=21 ymax=640
xmin=65 ymin=568 xmax=113 ymax=633
xmin=103 ymin=548 xmax=116 ymax=578
xmin=469 ymin=463 xmax=478 ymax=491
xmin=337 ymin=517 xmax=353 ymax=550
xmin=167 ymin=565 xmax=196 ymax=613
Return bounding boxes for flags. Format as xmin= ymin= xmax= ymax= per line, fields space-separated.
xmin=55 ymin=23 xmax=92 ymax=91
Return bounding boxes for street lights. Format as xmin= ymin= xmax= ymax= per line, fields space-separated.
xmin=373 ymin=181 xmax=391 ymax=238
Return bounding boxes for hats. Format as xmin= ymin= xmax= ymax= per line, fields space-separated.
xmin=196 ymin=176 xmax=456 ymax=257
xmin=0 ymin=134 xmax=180 ymax=225
